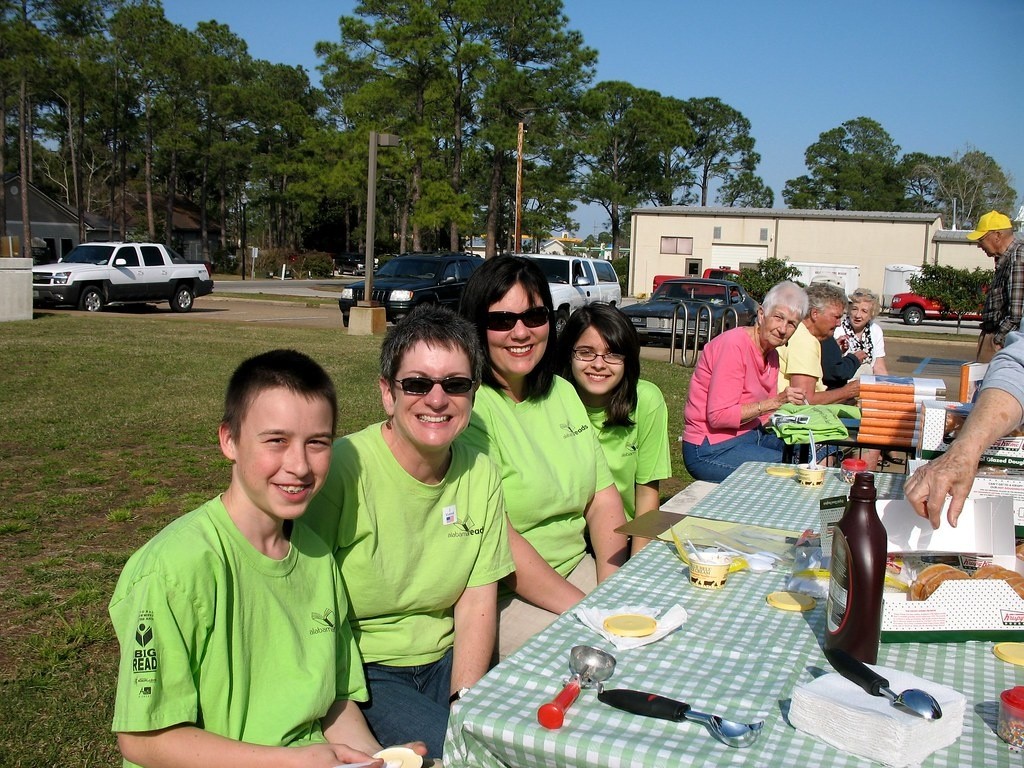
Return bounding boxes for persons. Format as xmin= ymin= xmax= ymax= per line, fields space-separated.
xmin=966 ymin=211 xmax=1024 ymax=403
xmin=775 ymin=283 xmax=906 ymax=469
xmin=298 ymin=306 xmax=517 ymax=768
xmin=459 ymin=255 xmax=631 ymax=665
xmin=683 ymin=281 xmax=840 ymax=485
xmin=556 ymin=301 xmax=672 ymax=564
xmin=902 ymin=319 xmax=1024 ymax=529
xmin=107 ymin=346 xmax=429 ymax=768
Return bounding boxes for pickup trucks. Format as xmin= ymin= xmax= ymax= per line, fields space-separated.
xmin=650 ymin=265 xmax=749 ymax=300
xmin=32 ymin=242 xmax=214 ymax=312
xmin=889 ymin=283 xmax=989 ymax=326
xmin=513 ymin=253 xmax=621 ymax=339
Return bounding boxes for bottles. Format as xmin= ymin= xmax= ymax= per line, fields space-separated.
xmin=825 ymin=472 xmax=887 ymax=666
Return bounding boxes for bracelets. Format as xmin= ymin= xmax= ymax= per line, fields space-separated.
xmin=757 ymin=402 xmax=762 ymax=417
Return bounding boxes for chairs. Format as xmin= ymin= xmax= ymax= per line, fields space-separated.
xmin=672 ymin=287 xmax=691 ymax=300
xmin=416 ymin=261 xmax=436 ymax=276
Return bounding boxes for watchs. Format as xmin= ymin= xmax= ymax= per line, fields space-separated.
xmin=449 ymin=687 xmax=471 ymax=705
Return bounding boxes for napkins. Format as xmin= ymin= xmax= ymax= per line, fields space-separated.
xmin=574 ymin=603 xmax=690 ymax=652
xmin=787 ymin=661 xmax=966 ymax=768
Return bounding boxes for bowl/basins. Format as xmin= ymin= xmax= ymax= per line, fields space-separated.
xmin=796 ymin=463 xmax=827 ymax=485
xmin=840 ymin=458 xmax=868 ymax=483
xmin=688 ymin=551 xmax=733 ymax=590
xmin=997 ymin=686 xmax=1024 ymax=748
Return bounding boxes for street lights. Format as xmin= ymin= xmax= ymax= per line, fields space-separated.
xmin=241 ymin=194 xmax=248 ymax=281
xmin=609 ymin=235 xmax=613 ymax=249
xmin=357 ymin=128 xmax=400 ymax=305
xmin=706 ymin=165 xmax=742 ymax=206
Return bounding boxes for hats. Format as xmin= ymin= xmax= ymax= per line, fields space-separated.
xmin=966 ymin=210 xmax=1012 ymax=240
xmin=809 ymin=275 xmax=853 ymax=303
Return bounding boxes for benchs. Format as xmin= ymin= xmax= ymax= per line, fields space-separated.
xmin=657 ymin=478 xmax=720 ymax=514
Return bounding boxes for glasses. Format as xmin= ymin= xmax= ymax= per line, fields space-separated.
xmin=392 ymin=377 xmax=476 ymax=394
xmin=572 ymin=348 xmax=626 ymax=364
xmin=978 ymin=232 xmax=997 ymax=243
xmin=857 ymin=292 xmax=876 ymax=299
xmin=486 ymin=305 xmax=550 ymax=330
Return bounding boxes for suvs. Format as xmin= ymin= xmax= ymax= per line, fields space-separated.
xmin=338 ymin=251 xmax=487 ymax=328
xmin=338 ymin=254 xmax=379 ymax=275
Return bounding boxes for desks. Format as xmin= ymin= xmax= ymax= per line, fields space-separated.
xmin=766 ymin=403 xmax=960 ymax=475
xmin=443 ymin=460 xmax=1024 ymax=768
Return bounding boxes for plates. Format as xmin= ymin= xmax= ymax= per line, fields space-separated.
xmin=372 ymin=746 xmax=423 ymax=768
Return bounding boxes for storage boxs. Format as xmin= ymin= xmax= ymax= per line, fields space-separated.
xmin=873 ymin=494 xmax=1024 ymax=644
xmin=915 ymin=399 xmax=1024 ymax=470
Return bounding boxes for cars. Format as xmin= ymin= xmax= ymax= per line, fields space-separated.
xmin=620 ymin=276 xmax=760 ymax=350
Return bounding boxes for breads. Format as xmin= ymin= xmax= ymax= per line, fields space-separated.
xmin=1015 ymin=543 xmax=1024 ymax=563
xmin=909 ymin=564 xmax=1024 ymax=601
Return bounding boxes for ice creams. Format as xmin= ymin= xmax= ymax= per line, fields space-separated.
xmin=696 ymin=559 xmax=729 ymax=565
xmin=803 ymin=465 xmax=825 ymax=470
xmin=374 ymin=750 xmax=422 ymax=768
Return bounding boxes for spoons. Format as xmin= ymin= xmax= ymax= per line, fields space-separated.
xmin=332 ymin=758 xmax=403 ymax=768
xmin=714 ymin=541 xmax=775 ymax=573
xmin=598 ymin=685 xmax=765 ymax=747
xmin=824 ymin=646 xmax=944 ymax=719
